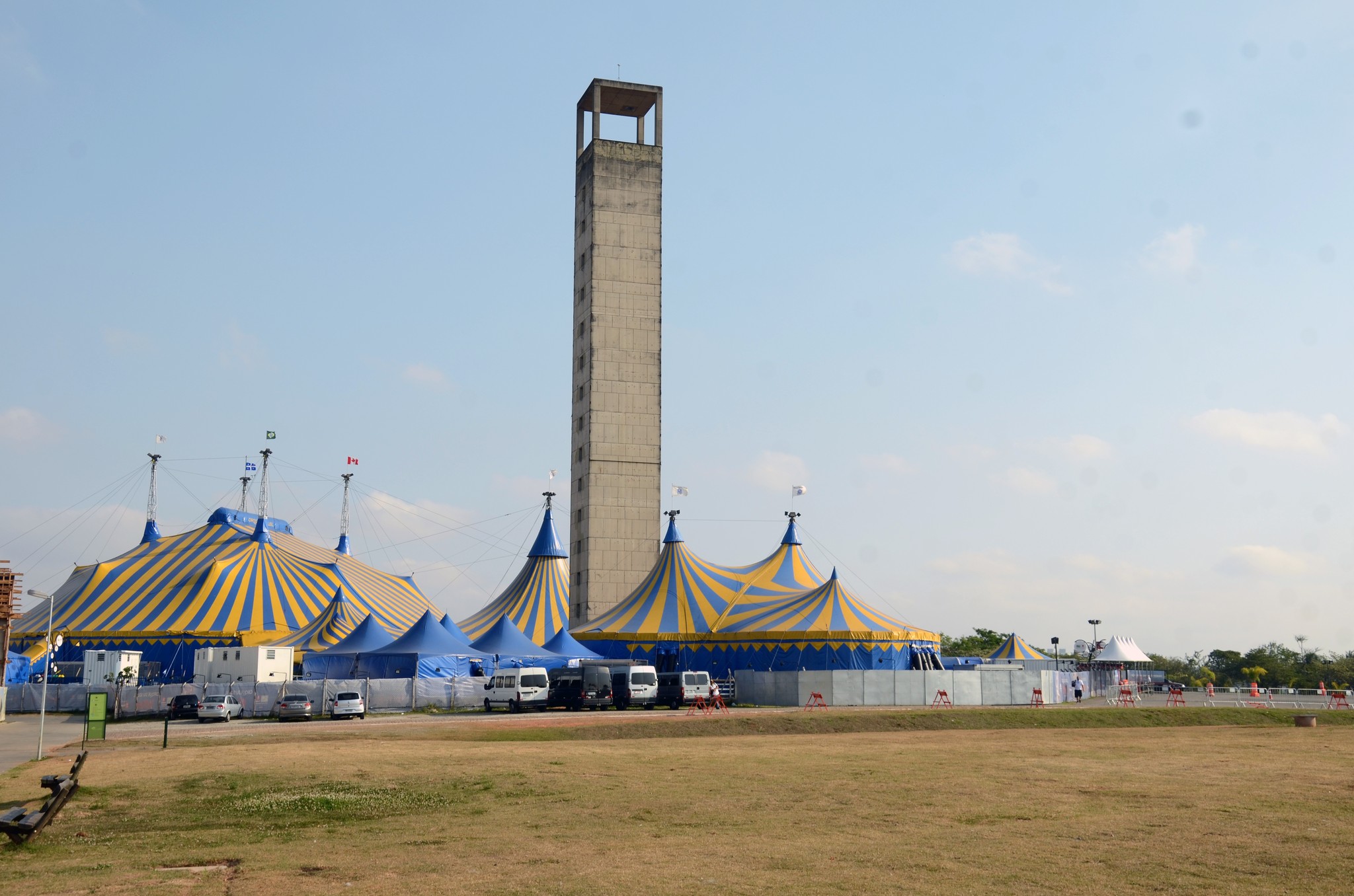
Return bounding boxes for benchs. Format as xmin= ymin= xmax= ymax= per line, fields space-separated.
xmin=0 ymin=778 xmax=78 ymax=847
xmin=39 ymin=749 xmax=89 ymax=801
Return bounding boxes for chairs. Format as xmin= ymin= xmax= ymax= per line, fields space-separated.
xmin=340 ymin=695 xmax=344 ymax=700
xmin=219 ymin=699 xmax=224 ymax=702
xmin=210 ymin=699 xmax=216 ymax=702
xmin=351 ymin=694 xmax=356 ymax=699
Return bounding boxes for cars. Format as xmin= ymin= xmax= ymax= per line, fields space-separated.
xmin=197 ymin=695 xmax=244 ymax=723
xmin=276 ymin=694 xmax=315 ymax=722
xmin=1140 ymin=678 xmax=1185 ymax=692
xmin=167 ymin=694 xmax=201 ymax=720
xmin=328 ymin=691 xmax=365 ymax=719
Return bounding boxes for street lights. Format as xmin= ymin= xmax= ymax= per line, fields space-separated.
xmin=1268 ymin=689 xmax=1273 ymax=700
xmin=1322 ymin=660 xmax=1333 ymax=695
xmin=27 ymin=589 xmax=55 ymax=762
xmin=1088 ymin=620 xmax=1102 ymax=658
xmin=1051 ymin=637 xmax=1059 ymax=671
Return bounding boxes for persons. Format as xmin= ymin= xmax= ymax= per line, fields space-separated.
xmin=710 ymin=679 xmax=720 ymax=709
xmin=1074 ymin=676 xmax=1085 ymax=704
xmin=1146 ymin=675 xmax=1151 ymax=683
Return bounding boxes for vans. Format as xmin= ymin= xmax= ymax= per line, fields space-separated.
xmin=655 ymin=670 xmax=711 ymax=710
xmin=484 ymin=667 xmax=551 ymax=713
xmin=546 ymin=665 xmax=613 ymax=712
xmin=608 ymin=666 xmax=659 ymax=711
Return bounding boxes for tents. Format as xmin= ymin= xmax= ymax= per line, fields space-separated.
xmin=2 ymin=451 xmax=1151 ymax=699
